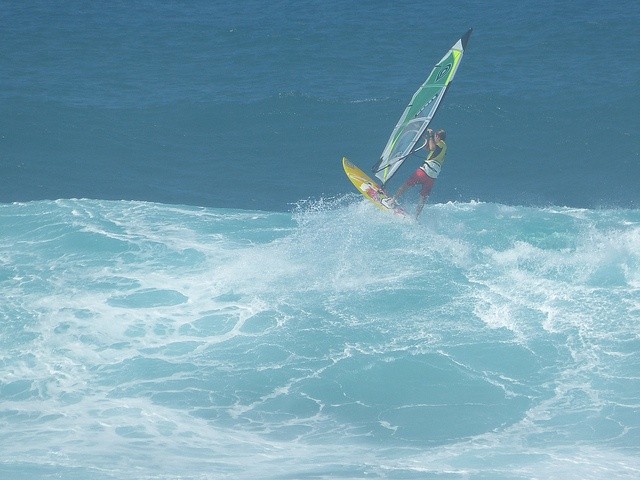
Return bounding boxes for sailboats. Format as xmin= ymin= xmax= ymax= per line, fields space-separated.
xmin=342 ymin=28 xmax=472 ymax=221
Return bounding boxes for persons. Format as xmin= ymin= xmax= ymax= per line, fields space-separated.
xmin=380 ymin=127 xmax=447 ymax=219
